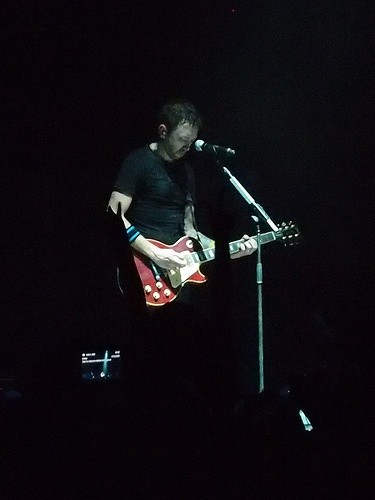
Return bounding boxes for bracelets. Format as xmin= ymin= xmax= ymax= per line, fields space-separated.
xmin=125 ymin=225 xmax=141 ymax=243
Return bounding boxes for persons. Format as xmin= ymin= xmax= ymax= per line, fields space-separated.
xmin=105 ymin=101 xmax=258 ymax=318
xmin=0 ymin=185 xmax=375 ymax=500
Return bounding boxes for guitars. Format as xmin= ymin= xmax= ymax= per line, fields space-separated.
xmin=117 ymin=225 xmax=300 ymax=307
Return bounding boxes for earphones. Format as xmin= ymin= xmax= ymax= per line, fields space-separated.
xmin=163 ymin=131 xmax=166 ymax=135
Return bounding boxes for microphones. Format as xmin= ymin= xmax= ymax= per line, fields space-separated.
xmin=194 ymin=140 xmax=235 ymax=155
